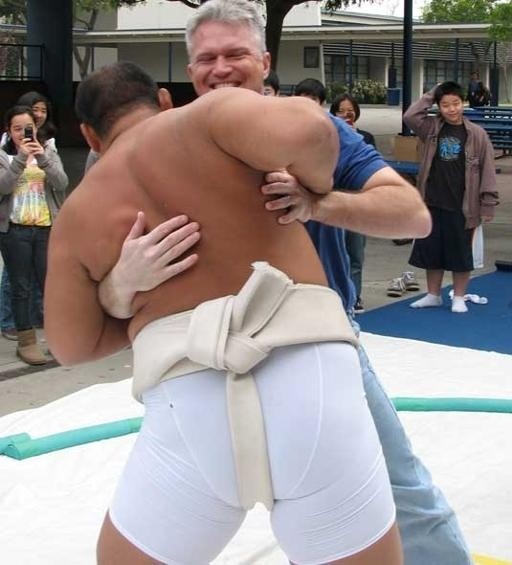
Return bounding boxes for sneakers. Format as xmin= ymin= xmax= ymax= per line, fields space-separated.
xmin=402 ymin=271 xmax=420 ymax=292
xmin=354 ymin=303 xmax=365 ymax=314
xmin=2 ymin=324 xmax=20 ymax=341
xmin=384 ymin=275 xmax=407 ymax=297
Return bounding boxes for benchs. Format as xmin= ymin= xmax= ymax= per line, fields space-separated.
xmin=427 ymin=105 xmax=512 ymax=159
xmin=383 ymin=132 xmax=425 ymax=185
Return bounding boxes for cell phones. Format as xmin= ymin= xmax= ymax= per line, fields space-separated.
xmin=345 ymin=118 xmax=353 ymax=125
xmin=25 ymin=127 xmax=34 ymax=144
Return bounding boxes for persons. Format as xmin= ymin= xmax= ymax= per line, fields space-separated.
xmin=0 ymin=105 xmax=70 ymax=367
xmin=467 ymin=71 xmax=494 ymax=109
xmin=0 ymin=90 xmax=57 ymax=342
xmin=96 ymin=0 xmax=474 ymax=565
xmin=401 ymin=80 xmax=497 ymax=314
xmin=295 ymin=77 xmax=326 ymax=109
xmin=38 ymin=61 xmax=404 ymax=564
xmin=259 ymin=67 xmax=279 ymax=97
xmin=330 ymin=94 xmax=376 ymax=314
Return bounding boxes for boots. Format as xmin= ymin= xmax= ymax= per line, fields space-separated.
xmin=15 ymin=326 xmax=49 ymax=366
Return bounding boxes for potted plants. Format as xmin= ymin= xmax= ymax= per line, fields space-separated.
xmin=352 ymin=79 xmax=387 ymax=104
